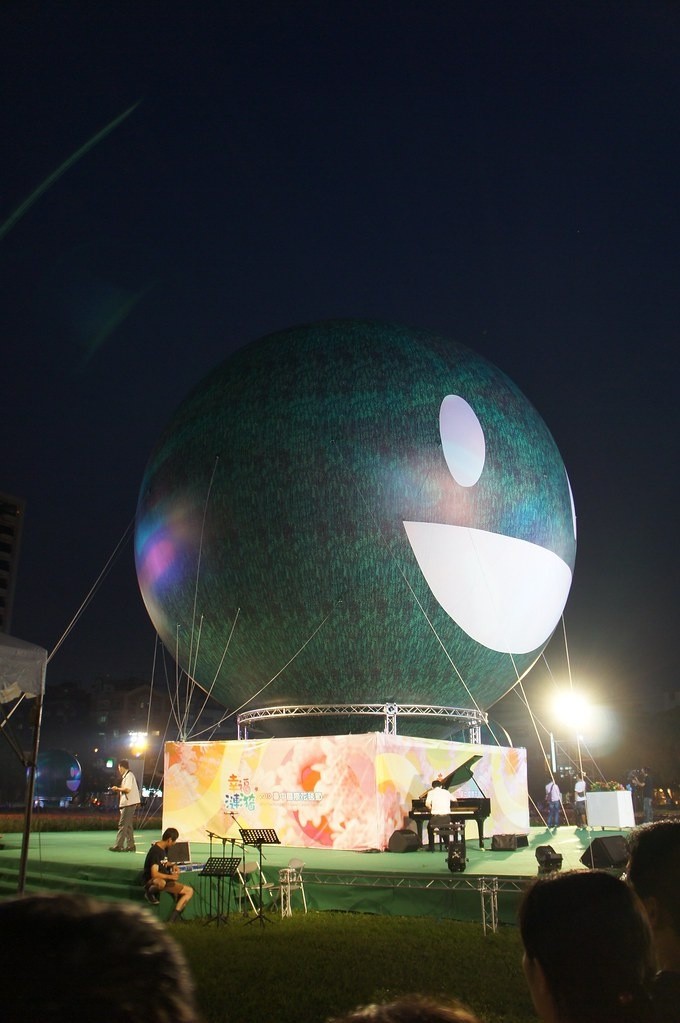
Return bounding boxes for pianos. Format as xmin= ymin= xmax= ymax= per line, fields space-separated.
xmin=407 ymin=754 xmax=493 ymax=849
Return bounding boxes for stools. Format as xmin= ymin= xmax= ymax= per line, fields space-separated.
xmin=430 ymin=825 xmax=465 ymax=853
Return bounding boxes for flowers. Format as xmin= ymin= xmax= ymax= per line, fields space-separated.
xmin=590 ymin=781 xmax=625 ymax=791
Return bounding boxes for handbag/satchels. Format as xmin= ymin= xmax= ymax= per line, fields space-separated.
xmin=544 ymin=783 xmax=554 ymax=803
xmin=578 ymin=791 xmax=586 ymax=797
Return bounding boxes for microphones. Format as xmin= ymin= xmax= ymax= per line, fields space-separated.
xmin=225 ymin=812 xmax=239 ymax=814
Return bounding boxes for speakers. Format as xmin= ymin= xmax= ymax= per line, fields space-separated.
xmin=491 ymin=834 xmax=517 ymax=851
xmin=579 ymin=835 xmax=630 ymax=869
xmin=388 ymin=829 xmax=421 ymax=853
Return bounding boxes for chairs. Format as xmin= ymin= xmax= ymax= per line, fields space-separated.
xmin=235 ymin=860 xmax=280 ymax=916
xmin=266 ymin=857 xmax=308 ymax=919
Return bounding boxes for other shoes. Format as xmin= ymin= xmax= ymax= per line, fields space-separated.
xmin=144 ymin=889 xmax=159 ymax=904
xmin=109 ymin=845 xmax=124 ymax=851
xmin=426 ymin=845 xmax=435 ymax=851
xmin=445 ymin=845 xmax=449 ymax=851
xmin=169 ymin=916 xmax=192 ymax=924
xmin=121 ymin=845 xmax=136 ymax=852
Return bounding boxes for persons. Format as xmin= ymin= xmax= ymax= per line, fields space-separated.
xmin=545 ymin=773 xmax=560 ymax=827
xmin=622 ymin=820 xmax=680 ymax=1023
xmin=142 ymin=828 xmax=193 ymax=922
xmin=518 ymin=868 xmax=658 ymax=1023
xmin=0 ymin=892 xmax=196 ymax=1023
xmin=632 ymin=766 xmax=654 ymax=824
xmin=425 ymin=780 xmax=458 ymax=852
xmin=573 ymin=773 xmax=587 ymax=824
xmin=107 ymin=759 xmax=140 ymax=852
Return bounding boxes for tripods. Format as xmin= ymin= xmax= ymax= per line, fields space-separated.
xmin=199 ymin=815 xmax=280 ymax=929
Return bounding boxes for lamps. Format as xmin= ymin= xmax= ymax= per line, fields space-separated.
xmin=443 ymin=849 xmax=469 ymax=873
xmin=536 ymin=845 xmax=563 ymax=874
xmin=577 ymin=834 xmax=630 ymax=870
